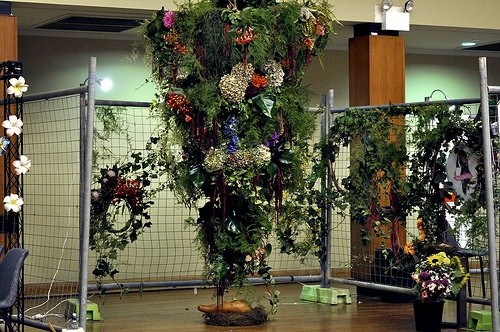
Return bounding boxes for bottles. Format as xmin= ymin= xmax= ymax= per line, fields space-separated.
xmin=68 ymin=313 xmax=78 ymax=330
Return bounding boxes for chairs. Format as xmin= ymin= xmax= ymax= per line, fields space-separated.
xmin=0 ymin=248 xmax=29 ymax=332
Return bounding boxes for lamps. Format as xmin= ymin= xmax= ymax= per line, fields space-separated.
xmin=425 ymin=90 xmax=455 ymax=112
xmin=80 ymin=78 xmax=112 ymax=91
xmin=381 ymin=0 xmax=414 ymax=31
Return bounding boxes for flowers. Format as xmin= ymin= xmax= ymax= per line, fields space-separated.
xmin=3 ymin=193 xmax=24 ymax=212
xmin=1 ymin=115 xmax=24 ymax=137
xmin=6 ymin=76 xmax=29 ymax=98
xmin=12 ymin=154 xmax=31 ymax=175
xmin=403 ymin=217 xmax=470 ymax=301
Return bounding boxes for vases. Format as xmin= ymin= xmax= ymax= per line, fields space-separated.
xmin=413 ymin=300 xmax=445 ymax=332
